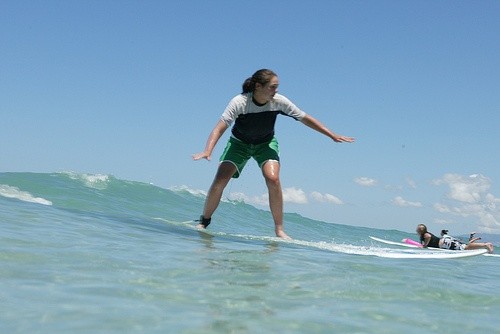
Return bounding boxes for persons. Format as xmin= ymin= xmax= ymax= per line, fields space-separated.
xmin=440 ymin=229 xmax=481 ymax=244
xmin=416 ymin=224 xmax=493 ymax=254
xmin=192 ymin=69 xmax=355 ymax=241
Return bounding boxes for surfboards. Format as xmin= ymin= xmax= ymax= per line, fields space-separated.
xmin=152 ymin=216 xmax=488 ymax=259
xmin=482 ymin=253 xmax=500 ymax=258
xmin=369 ymin=234 xmax=468 ymax=250
